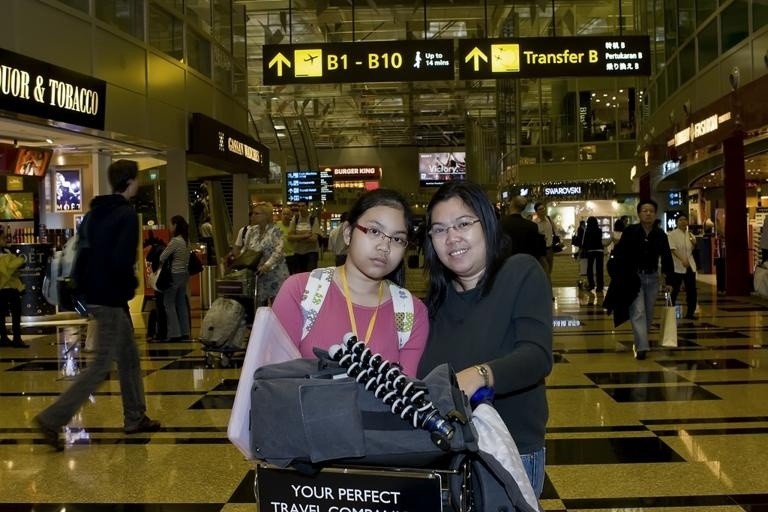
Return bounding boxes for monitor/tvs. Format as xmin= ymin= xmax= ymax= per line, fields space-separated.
xmin=418 ymin=151 xmax=466 ymax=185
xmin=13 ymin=146 xmax=53 ymax=179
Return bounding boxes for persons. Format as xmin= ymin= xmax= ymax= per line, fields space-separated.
xmin=582 ymin=216 xmax=604 ymax=292
xmin=220 ymin=198 xmax=324 ymax=309
xmin=609 ymin=198 xmax=674 ymax=361
xmin=668 ymin=213 xmax=698 ymax=320
xmin=574 ymin=221 xmax=586 ymax=260
xmin=258 ymin=188 xmax=430 ymax=473
xmin=407 ymin=223 xmax=426 ymax=256
xmin=32 ymin=161 xmax=162 ymax=452
xmin=621 ymin=216 xmax=629 ymax=230
xmin=159 ymin=215 xmax=190 ymax=342
xmin=655 ymin=218 xmax=661 ymax=231
xmin=56 ymin=182 xmax=80 ymax=210
xmin=492 ymin=195 xmax=556 ymax=302
xmin=613 ymin=219 xmax=625 ymax=246
xmin=416 ymin=180 xmax=553 ymax=500
xmin=3 ymin=236 xmax=30 ymax=348
xmin=328 ymin=212 xmax=353 ymax=268
xmin=199 ymin=216 xmax=214 ymax=247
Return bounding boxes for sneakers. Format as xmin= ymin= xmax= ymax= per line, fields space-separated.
xmin=12 ymin=340 xmax=31 ymax=348
xmin=0 ymin=338 xmax=12 ymax=347
xmin=123 ymin=415 xmax=162 ymax=433
xmin=32 ymin=415 xmax=65 ymax=452
xmin=636 ymin=351 xmax=646 ymax=360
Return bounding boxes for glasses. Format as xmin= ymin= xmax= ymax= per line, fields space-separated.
xmin=427 ymin=214 xmax=480 ymax=236
xmin=351 ymin=222 xmax=410 ymax=249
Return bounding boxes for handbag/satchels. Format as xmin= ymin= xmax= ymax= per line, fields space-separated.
xmin=552 ymin=236 xmax=563 ymax=253
xmin=657 ymin=293 xmax=679 ymax=348
xmin=248 ymin=346 xmax=480 ymax=477
xmin=188 ymin=252 xmax=204 ymax=276
xmin=229 ymin=248 xmax=264 ymax=272
xmin=197 ymin=296 xmax=247 ymax=349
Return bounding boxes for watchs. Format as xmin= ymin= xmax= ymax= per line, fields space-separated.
xmin=475 ymin=365 xmax=489 ymax=386
